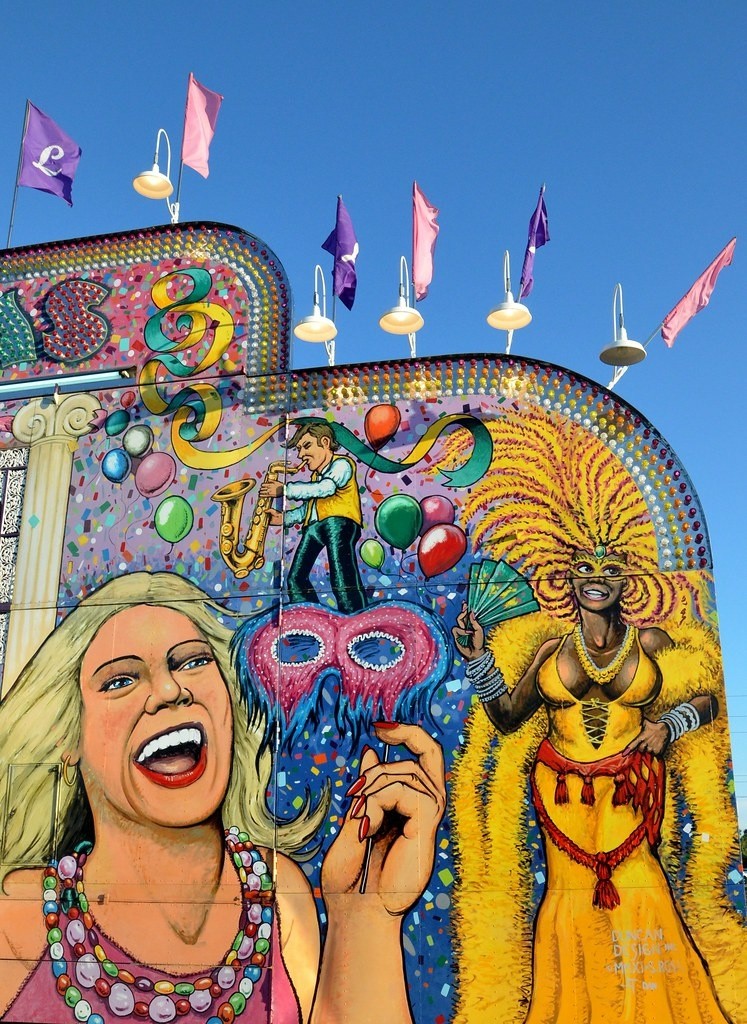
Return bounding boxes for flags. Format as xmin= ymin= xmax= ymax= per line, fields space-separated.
xmin=321 ymin=196 xmax=361 ymax=311
xmin=15 ymin=99 xmax=83 ymax=207
xmin=518 ymin=187 xmax=550 ymax=298
xmin=411 ymin=182 xmax=440 ymax=302
xmin=180 ymin=73 xmax=225 ymax=179
xmin=662 ymin=238 xmax=736 ymax=348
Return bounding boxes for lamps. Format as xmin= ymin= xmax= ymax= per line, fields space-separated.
xmin=486 ymin=249 xmax=532 ymax=354
xmin=133 ymin=129 xmax=179 ymax=224
xmin=379 ymin=256 xmax=424 ymax=358
xmin=294 ymin=265 xmax=338 ymax=366
xmin=599 ymin=282 xmax=646 ymax=382
